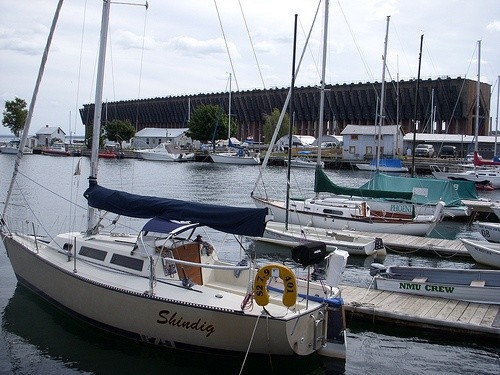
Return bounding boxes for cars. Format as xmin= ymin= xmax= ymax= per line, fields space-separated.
xmin=440 ymin=145 xmax=459 ymax=159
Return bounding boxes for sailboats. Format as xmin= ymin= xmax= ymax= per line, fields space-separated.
xmin=0 ymin=0 xmax=348 ymax=362
xmin=208 ymin=73 xmax=261 ymax=165
xmin=250 ymin=0 xmax=500 ymax=268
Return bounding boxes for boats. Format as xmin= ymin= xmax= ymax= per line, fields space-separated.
xmin=132 ymin=143 xmax=188 ymax=161
xmin=99 ymin=145 xmax=124 ymax=159
xmin=42 ymin=141 xmax=74 ymax=158
xmin=369 ymin=261 xmax=500 ymax=305
xmin=0 ymin=140 xmax=33 ymax=155
xmin=355 ymin=158 xmax=408 ymax=172
xmin=284 ymin=150 xmax=325 ymax=168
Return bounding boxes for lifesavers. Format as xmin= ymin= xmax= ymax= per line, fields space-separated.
xmin=253 ymin=262 xmax=297 ymax=308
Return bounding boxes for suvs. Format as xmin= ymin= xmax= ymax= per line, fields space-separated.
xmin=414 ymin=144 xmax=435 ymax=158
